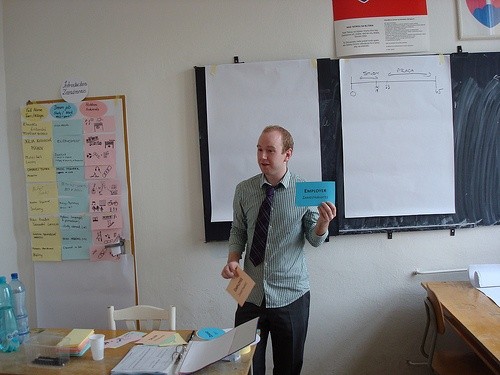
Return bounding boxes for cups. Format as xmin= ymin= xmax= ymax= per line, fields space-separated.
xmin=88 ymin=334 xmax=105 ymax=361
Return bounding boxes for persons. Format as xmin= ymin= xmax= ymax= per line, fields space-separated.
xmin=221 ymin=125 xmax=336 ymax=375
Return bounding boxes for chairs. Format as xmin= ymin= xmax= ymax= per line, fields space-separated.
xmin=407 ymin=288 xmax=493 ymax=375
xmin=106 ymin=304 xmax=176 ymax=330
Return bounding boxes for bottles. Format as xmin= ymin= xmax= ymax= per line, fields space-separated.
xmin=0 ymin=275 xmax=20 ymax=352
xmin=7 ymin=273 xmax=30 ymax=345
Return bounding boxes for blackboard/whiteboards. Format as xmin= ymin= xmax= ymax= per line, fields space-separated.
xmin=195 ymin=52 xmax=500 ymax=243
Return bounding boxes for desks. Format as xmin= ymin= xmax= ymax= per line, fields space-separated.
xmin=0 ymin=327 xmax=260 ymax=375
xmin=421 ymin=281 xmax=500 ymax=375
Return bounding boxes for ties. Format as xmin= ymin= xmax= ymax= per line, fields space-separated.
xmin=248 ymin=182 xmax=282 ymax=267
